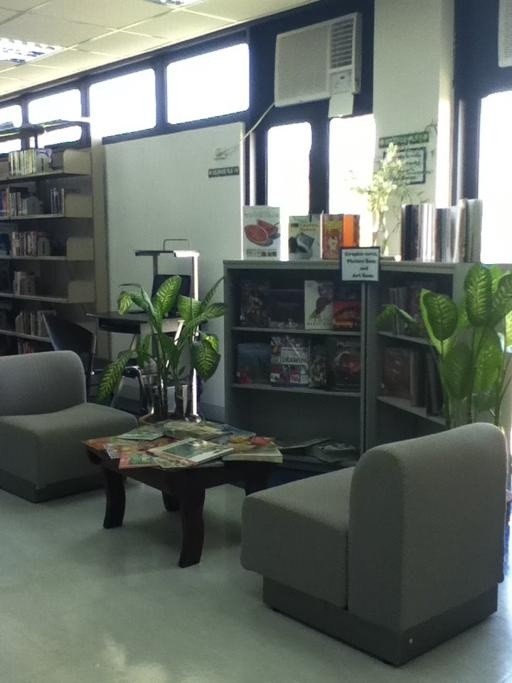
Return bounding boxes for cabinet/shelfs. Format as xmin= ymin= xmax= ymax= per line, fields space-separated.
xmin=365 ymin=262 xmax=512 ymax=452
xmin=223 ymin=259 xmax=365 ymax=472
xmin=0 ymin=114 xmax=111 ymax=404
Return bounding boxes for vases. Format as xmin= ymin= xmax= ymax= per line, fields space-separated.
xmin=372 ymin=231 xmax=386 ymax=257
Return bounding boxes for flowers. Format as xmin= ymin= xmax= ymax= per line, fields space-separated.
xmin=348 ymin=141 xmax=419 ymax=231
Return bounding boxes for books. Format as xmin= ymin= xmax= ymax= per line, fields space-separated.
xmin=382 ymin=198 xmax=483 ymax=417
xmin=0 ymin=148 xmax=66 ymax=354
xmin=83 ymin=417 xmax=358 ymax=469
xmin=236 ymin=206 xmax=358 ymax=393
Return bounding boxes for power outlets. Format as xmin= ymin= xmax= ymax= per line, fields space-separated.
xmin=213 ymin=145 xmax=225 ymax=159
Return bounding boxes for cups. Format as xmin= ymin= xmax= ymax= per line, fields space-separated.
xmin=400 ymin=198 xmax=483 ymax=265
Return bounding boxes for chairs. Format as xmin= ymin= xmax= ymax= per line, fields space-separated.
xmin=240 ymin=421 xmax=506 ymax=668
xmin=0 ymin=350 xmax=140 ymax=503
